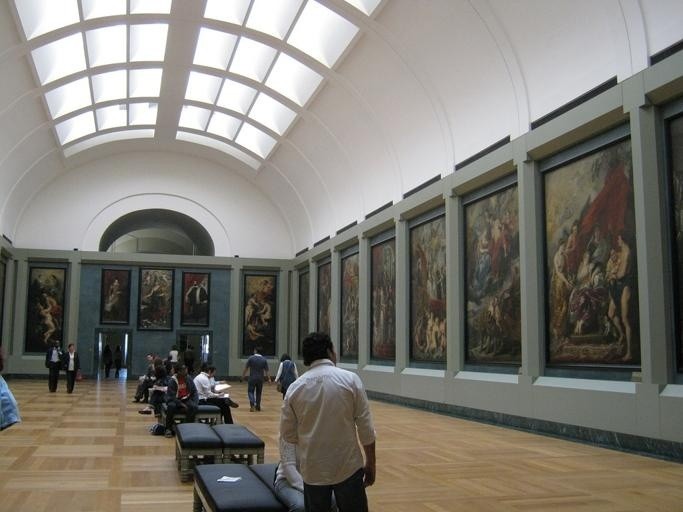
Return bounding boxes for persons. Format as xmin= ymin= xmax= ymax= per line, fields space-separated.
xmin=140 ymin=271 xmax=171 ymax=325
xmin=0 ymin=374 xmax=22 ymax=435
xmin=246 ymin=280 xmax=276 ymax=357
xmin=105 ymin=277 xmax=123 ymax=313
xmin=102 ymin=344 xmax=122 ymax=378
xmin=46 ymin=343 xmax=61 ymax=392
xmin=186 ymin=279 xmax=208 ymax=319
xmin=132 ymin=343 xmax=239 ymax=433
xmin=62 ymin=344 xmax=80 ymax=393
xmin=280 ymin=331 xmax=376 ymax=511
xmin=275 ymin=354 xmax=298 ymax=401
xmin=30 ymin=270 xmax=62 ymax=342
xmin=240 ymin=347 xmax=271 ymax=412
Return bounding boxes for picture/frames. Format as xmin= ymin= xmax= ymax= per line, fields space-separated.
xmin=99 ymin=267 xmax=132 ymax=327
xmin=299 ymin=271 xmax=309 ymax=360
xmin=241 ymin=273 xmax=277 ymax=357
xmin=24 ymin=266 xmax=66 ymax=354
xmin=539 ymin=132 xmax=642 ymax=372
xmin=315 ymin=261 xmax=330 ymax=336
xmin=663 ymin=112 xmax=682 ymax=376
xmin=0 ymin=261 xmax=6 ymax=348
xmin=461 ymin=181 xmax=523 ymax=368
xmin=136 ymin=266 xmax=176 ymax=332
xmin=338 ymin=251 xmax=358 ymax=361
xmin=179 ymin=271 xmax=211 ymax=328
xmin=370 ymin=237 xmax=396 ymax=361
xmin=408 ymin=212 xmax=447 ymax=365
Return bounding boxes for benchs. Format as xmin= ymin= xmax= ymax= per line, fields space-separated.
xmin=247 ymin=464 xmax=340 ymax=512
xmin=190 ymin=463 xmax=287 ymax=511
xmin=173 ymin=421 xmax=225 ymax=483
xmin=139 ymin=377 xmax=224 ymax=436
xmin=212 ymin=423 xmax=265 ymax=466
